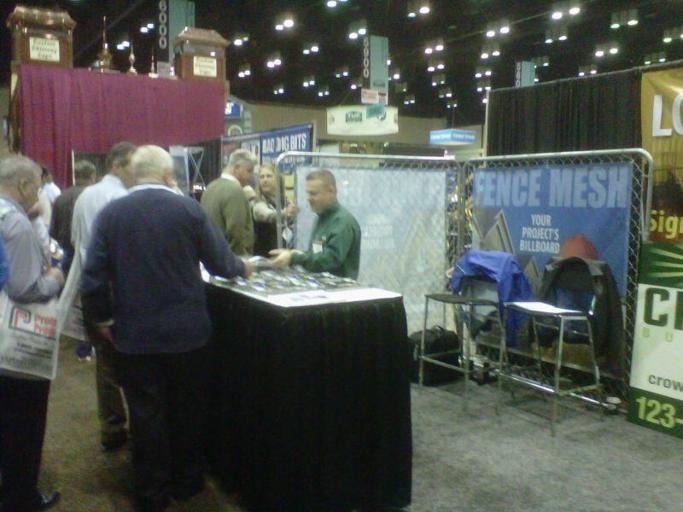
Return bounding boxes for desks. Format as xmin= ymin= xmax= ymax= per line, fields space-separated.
xmin=198 ymin=251 xmax=404 ymax=510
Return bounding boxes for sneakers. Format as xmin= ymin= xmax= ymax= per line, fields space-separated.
xmin=34 ymin=489 xmax=59 ymax=509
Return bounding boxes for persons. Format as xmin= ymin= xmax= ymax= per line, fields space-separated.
xmin=33 ymin=165 xmax=50 ymax=227
xmin=76 ymin=144 xmax=256 ymax=511
xmin=72 ymin=141 xmax=141 ymax=451
xmin=48 ymin=159 xmax=99 ymax=363
xmin=0 ymin=153 xmax=68 ymax=512
xmin=249 ymin=168 xmax=295 ymax=252
xmin=265 ymin=169 xmax=362 ymax=281
xmin=45 ymin=172 xmax=64 ymax=204
xmin=196 ymin=148 xmax=260 ymax=258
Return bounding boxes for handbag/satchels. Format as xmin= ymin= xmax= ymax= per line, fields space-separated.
xmin=0 ymin=247 xmax=66 ymax=382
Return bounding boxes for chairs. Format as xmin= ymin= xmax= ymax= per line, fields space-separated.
xmin=418 ymin=247 xmax=611 ymax=439
